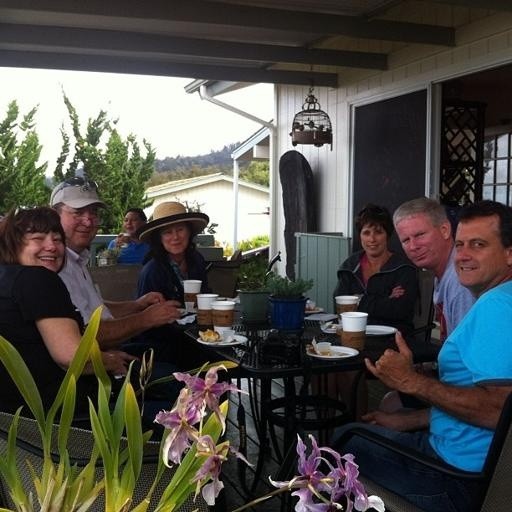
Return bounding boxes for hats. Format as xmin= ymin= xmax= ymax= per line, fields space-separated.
xmin=49 ymin=175 xmax=106 ymax=210
xmin=135 ymin=201 xmax=209 ymax=241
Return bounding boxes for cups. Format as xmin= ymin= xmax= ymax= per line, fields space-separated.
xmin=341 ymin=311 xmax=368 ymax=351
xmin=211 ymin=301 xmax=235 ymax=333
xmin=196 ymin=294 xmax=218 ymax=326
xmin=335 ymin=295 xmax=358 ymax=324
xmin=120 ymin=232 xmax=130 ymax=248
xmin=183 ymin=280 xmax=202 ymax=308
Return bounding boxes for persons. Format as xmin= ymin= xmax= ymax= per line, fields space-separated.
xmin=38 ymin=177 xmax=183 ymax=368
xmin=105 ymin=208 xmax=150 ymax=265
xmin=135 ymin=202 xmax=210 ymax=369
xmin=1 ymin=207 xmax=187 ymax=446
xmin=332 ymin=206 xmax=419 ymax=422
xmin=313 ymin=199 xmax=512 ymax=510
xmin=380 ymin=196 xmax=479 ymax=415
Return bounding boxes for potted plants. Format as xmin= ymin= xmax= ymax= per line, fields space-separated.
xmin=233 ymin=251 xmax=276 ymax=321
xmin=267 ymin=274 xmax=316 ymax=329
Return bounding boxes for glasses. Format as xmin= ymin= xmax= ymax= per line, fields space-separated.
xmin=10 ymin=202 xmax=38 ymax=222
xmin=48 ymin=176 xmax=100 ymax=206
xmin=60 ymin=209 xmax=104 ymax=226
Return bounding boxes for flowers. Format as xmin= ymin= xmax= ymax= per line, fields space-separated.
xmin=153 ymin=370 xmax=387 ymax=512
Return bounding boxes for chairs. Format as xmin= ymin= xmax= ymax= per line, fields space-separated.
xmin=0 ymin=410 xmax=208 ymax=512
xmin=346 ymin=391 xmax=512 ymax=512
xmin=205 ymin=260 xmax=239 ymax=296
xmin=88 ymin=262 xmax=147 ymax=303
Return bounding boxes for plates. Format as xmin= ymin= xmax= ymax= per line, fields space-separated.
xmin=305 ymin=307 xmax=324 ymax=314
xmin=365 ymin=324 xmax=396 ymax=336
xmin=322 ymin=320 xmax=341 ymax=334
xmin=197 ymin=334 xmax=248 ymax=347
xmin=306 ymin=345 xmax=359 ymax=360
